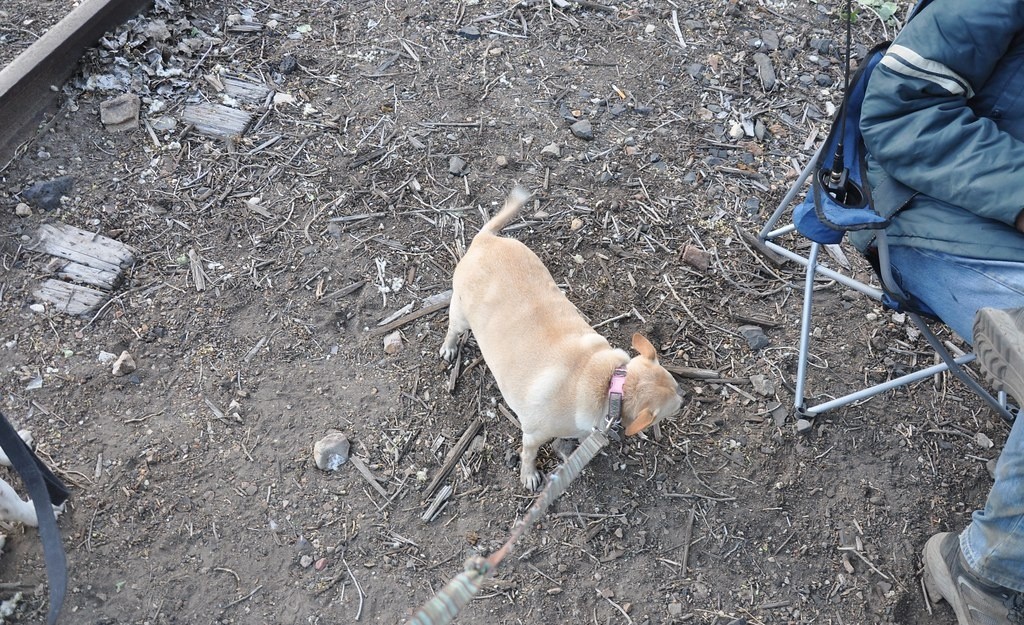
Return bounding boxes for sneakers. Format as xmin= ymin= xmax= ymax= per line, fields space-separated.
xmin=972 ymin=305 xmax=1024 ymax=411
xmin=922 ymin=532 xmax=1024 ymax=625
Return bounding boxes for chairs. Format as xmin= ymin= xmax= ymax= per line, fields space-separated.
xmin=758 ymin=0 xmax=1018 ymax=424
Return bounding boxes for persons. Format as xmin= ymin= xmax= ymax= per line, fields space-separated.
xmin=858 ymin=0 xmax=1024 ymax=625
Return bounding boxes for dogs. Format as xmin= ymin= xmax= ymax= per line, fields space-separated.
xmin=439 ymin=188 xmax=692 ymax=495
